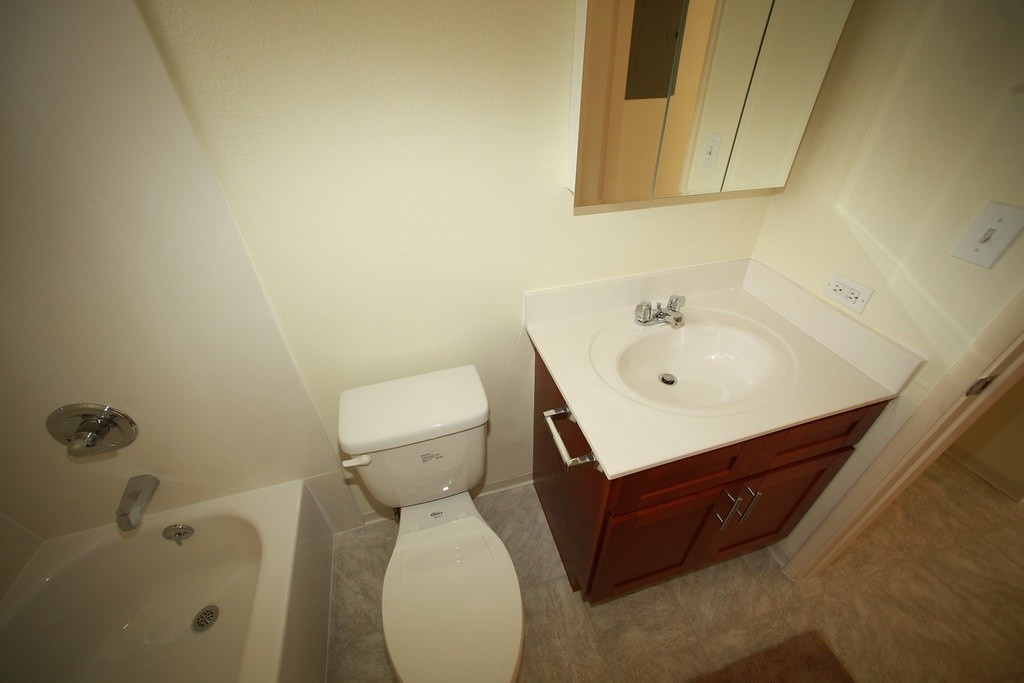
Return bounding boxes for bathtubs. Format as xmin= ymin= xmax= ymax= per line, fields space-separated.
xmin=0 ymin=480 xmax=334 ymax=683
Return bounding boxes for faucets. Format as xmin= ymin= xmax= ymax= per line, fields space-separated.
xmin=116 ymin=474 xmax=160 ymax=532
xmin=653 ymin=308 xmax=686 ymax=330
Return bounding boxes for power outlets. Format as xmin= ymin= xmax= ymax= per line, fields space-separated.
xmin=821 ymin=273 xmax=874 ymax=314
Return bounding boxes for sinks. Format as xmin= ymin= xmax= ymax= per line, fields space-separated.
xmin=589 ymin=305 xmax=797 ymax=417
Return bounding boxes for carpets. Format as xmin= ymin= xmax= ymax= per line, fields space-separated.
xmin=943 ymin=375 xmax=1024 ymax=506
xmin=683 ymin=629 xmax=859 ymax=683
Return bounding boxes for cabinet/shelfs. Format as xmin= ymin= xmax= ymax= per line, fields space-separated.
xmin=532 ymin=346 xmax=898 ymax=607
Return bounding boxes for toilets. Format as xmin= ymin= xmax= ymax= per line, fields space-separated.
xmin=336 ymin=364 xmax=524 ymax=682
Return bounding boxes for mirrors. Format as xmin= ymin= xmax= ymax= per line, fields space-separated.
xmin=567 ymin=1 xmax=854 ymax=219
